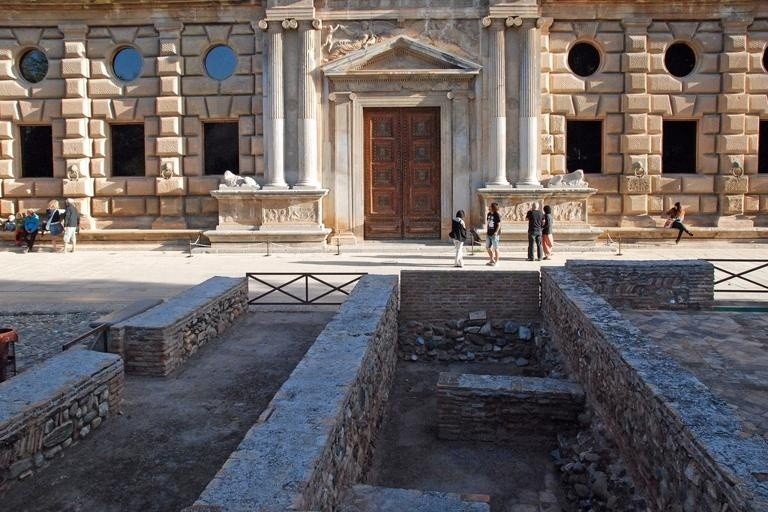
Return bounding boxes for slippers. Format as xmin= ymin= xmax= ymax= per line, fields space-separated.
xmin=485 ymin=261 xmax=498 ymax=266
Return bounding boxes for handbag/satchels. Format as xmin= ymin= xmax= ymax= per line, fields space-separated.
xmin=45 ymin=221 xmax=50 ymax=231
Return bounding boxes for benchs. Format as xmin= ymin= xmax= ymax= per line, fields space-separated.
xmin=90 ymin=298 xmax=164 ymax=352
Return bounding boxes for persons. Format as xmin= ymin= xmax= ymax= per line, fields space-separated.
xmin=340 ymin=20 xmax=378 ymax=49
xmin=449 ymin=209 xmax=467 ymax=268
xmin=0 ymin=196 xmax=80 ymax=254
xmin=540 ymin=205 xmax=553 ymax=260
xmin=665 ymin=202 xmax=695 ymax=245
xmin=525 ymin=201 xmax=546 ymax=261
xmin=485 ymin=202 xmax=502 ymax=266
xmin=321 ymin=24 xmax=340 ymax=55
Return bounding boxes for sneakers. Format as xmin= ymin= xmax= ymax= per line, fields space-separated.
xmin=526 ymin=257 xmax=550 ymax=261
